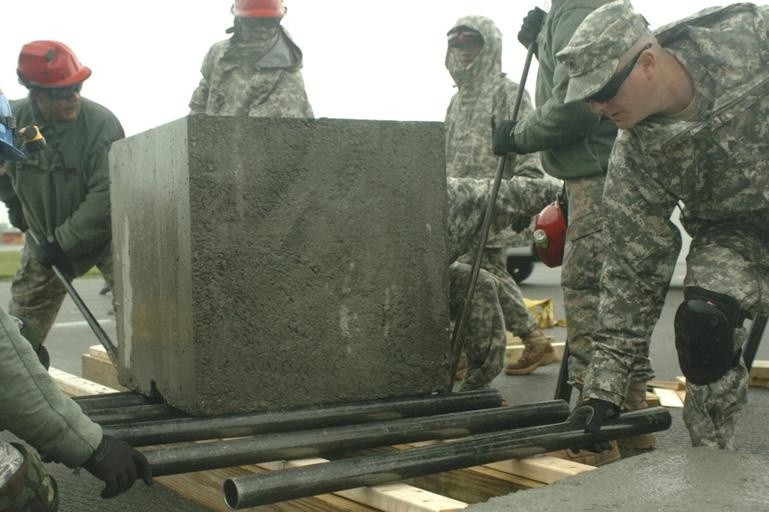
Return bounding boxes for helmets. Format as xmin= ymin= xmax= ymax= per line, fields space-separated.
xmin=231 ymin=0 xmax=284 ymax=17
xmin=17 ymin=40 xmax=91 ymax=89
xmin=534 ymin=201 xmax=566 ymax=267
xmin=0 ymin=91 xmax=28 ymax=162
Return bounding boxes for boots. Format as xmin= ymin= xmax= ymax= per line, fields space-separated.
xmin=548 ymin=383 xmax=657 ymax=467
xmin=504 ymin=328 xmax=556 ymax=375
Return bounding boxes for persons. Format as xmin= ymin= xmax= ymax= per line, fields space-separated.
xmin=0 ymin=305 xmax=157 ymax=512
xmin=0 ymin=39 xmax=130 ymax=364
xmin=186 ymin=0 xmax=318 ymax=121
xmin=442 ymin=175 xmax=569 ymax=397
xmin=489 ymin=1 xmax=659 ymax=465
xmin=440 ymin=14 xmax=560 ymax=376
xmin=558 ymin=0 xmax=768 ymax=456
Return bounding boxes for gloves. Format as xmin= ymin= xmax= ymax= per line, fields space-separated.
xmin=493 ymin=121 xmax=518 ymax=155
xmin=518 ymin=7 xmax=548 ymax=60
xmin=37 ymin=242 xmax=63 ymax=269
xmin=2 ymin=195 xmax=24 ymax=227
xmin=565 ymin=397 xmax=620 ymax=453
xmin=80 ymin=434 xmax=153 ymax=499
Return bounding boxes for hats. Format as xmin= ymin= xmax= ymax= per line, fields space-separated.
xmin=448 ymin=26 xmax=483 ymax=48
xmin=555 ymin=0 xmax=651 ymax=103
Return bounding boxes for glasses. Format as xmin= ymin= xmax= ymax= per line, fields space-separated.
xmin=585 ymin=43 xmax=652 ymax=103
xmin=31 ymin=82 xmax=82 ymax=99
